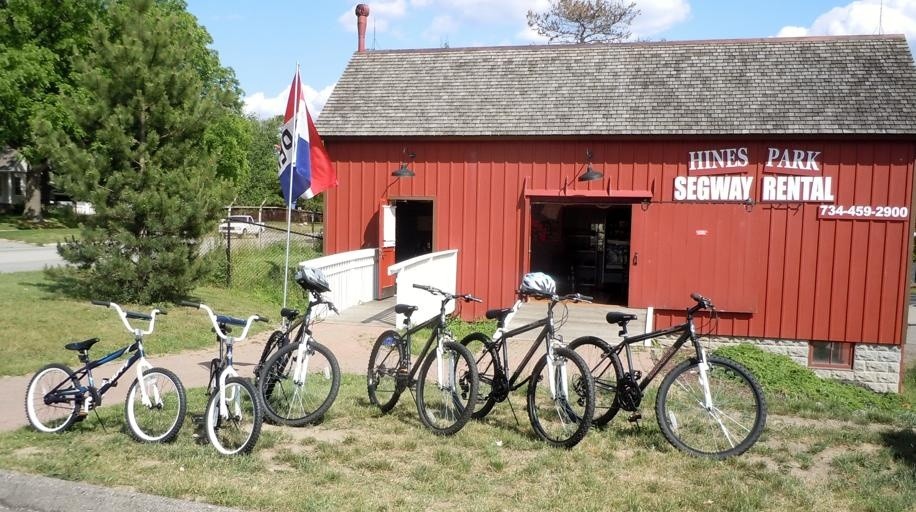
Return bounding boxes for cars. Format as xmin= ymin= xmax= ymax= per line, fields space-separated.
xmin=218 ymin=214 xmax=266 ymax=239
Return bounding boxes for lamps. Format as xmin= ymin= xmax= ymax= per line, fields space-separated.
xmin=391 ymin=160 xmax=415 ymax=177
xmin=577 ymin=150 xmax=603 ymax=181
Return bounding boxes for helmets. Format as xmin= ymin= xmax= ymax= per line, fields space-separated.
xmin=520 ymin=271 xmax=556 ymax=297
xmin=295 ymin=268 xmax=330 ymax=292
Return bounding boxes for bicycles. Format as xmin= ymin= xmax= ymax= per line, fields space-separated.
xmin=180 ymin=290 xmax=340 ymax=454
xmin=25 ymin=300 xmax=186 ymax=442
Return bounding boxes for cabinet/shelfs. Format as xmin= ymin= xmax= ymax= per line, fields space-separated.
xmin=567 ymin=230 xmax=599 ymax=287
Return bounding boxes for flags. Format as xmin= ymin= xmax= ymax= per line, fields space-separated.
xmin=279 ymin=72 xmax=337 ymax=206
